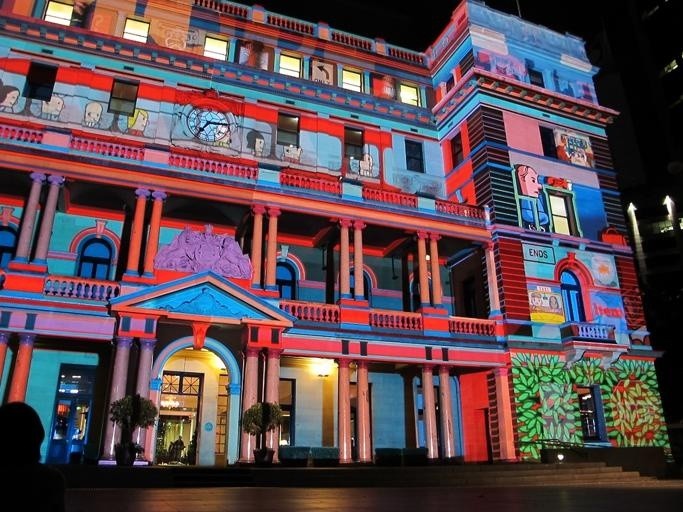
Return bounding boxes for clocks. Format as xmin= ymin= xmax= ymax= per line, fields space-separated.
xmin=186 ymin=104 xmax=230 ymax=142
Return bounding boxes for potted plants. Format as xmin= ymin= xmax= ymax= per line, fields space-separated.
xmin=243 ymin=402 xmax=282 ymax=466
xmin=106 ymin=393 xmax=157 ymax=469
